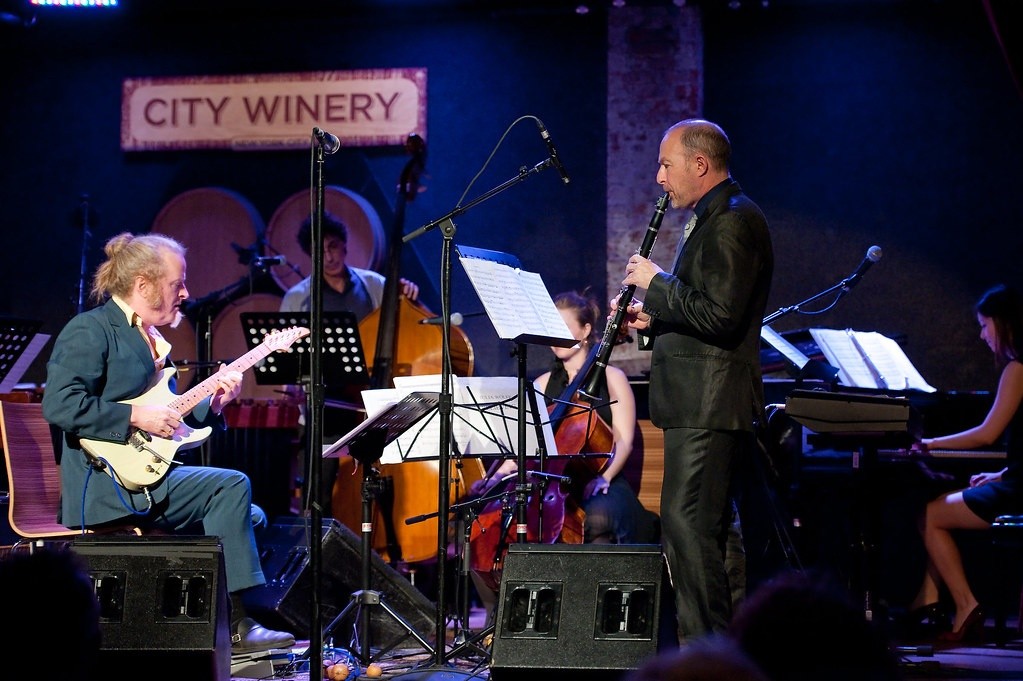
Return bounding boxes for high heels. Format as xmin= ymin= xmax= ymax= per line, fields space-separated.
xmin=938 ymin=603 xmax=990 ymax=644
xmin=891 ymin=598 xmax=940 ymax=635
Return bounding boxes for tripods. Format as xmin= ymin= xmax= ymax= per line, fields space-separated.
xmin=285 ymin=388 xmax=548 ymax=670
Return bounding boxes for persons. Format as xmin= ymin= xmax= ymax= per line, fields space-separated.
xmin=467 ymin=289 xmax=637 ymax=626
xmin=279 ymin=211 xmax=419 ymax=512
xmin=41 ymin=231 xmax=295 ymax=654
xmin=897 ymin=282 xmax=1023 ymax=645
xmin=607 ymin=120 xmax=773 ymax=649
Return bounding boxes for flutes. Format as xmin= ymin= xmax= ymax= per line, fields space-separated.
xmin=576 ymin=192 xmax=670 ymax=401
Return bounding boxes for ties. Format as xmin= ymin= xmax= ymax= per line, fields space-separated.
xmin=677 ymin=217 xmax=694 ymax=268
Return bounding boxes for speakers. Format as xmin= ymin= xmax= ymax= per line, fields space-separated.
xmin=490 ymin=542 xmax=679 ymax=681
xmin=66 ymin=534 xmax=232 ymax=681
xmin=250 ymin=518 xmax=439 ymax=651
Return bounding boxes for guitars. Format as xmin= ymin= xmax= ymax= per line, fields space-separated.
xmin=79 ymin=327 xmax=310 ymax=492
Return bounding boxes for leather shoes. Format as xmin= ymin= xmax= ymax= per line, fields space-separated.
xmin=230 ymin=616 xmax=296 ymax=654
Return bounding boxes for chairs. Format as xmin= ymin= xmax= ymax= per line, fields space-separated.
xmin=0 ymin=400 xmax=164 ymax=539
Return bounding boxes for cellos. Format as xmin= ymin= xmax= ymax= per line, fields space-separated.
xmin=330 ymin=133 xmax=487 ymax=586
xmin=460 ymin=326 xmax=633 ymax=664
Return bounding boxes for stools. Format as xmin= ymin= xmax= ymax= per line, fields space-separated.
xmin=988 ymin=515 xmax=1023 ymax=648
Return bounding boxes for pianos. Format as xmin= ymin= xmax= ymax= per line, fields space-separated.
xmin=627 ymin=325 xmax=1007 ymax=571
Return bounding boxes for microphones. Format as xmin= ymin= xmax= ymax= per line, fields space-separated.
xmin=841 ymin=245 xmax=884 ymax=292
xmin=313 ymin=126 xmax=340 ymax=154
xmin=421 ymin=312 xmax=463 ymax=325
xmin=256 ymin=255 xmax=286 ymax=266
xmin=537 ymin=119 xmax=571 ymax=184
xmin=526 ymin=471 xmax=572 ymax=486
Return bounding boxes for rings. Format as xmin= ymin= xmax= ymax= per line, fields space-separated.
xmin=167 ymin=426 xmax=172 ymax=434
